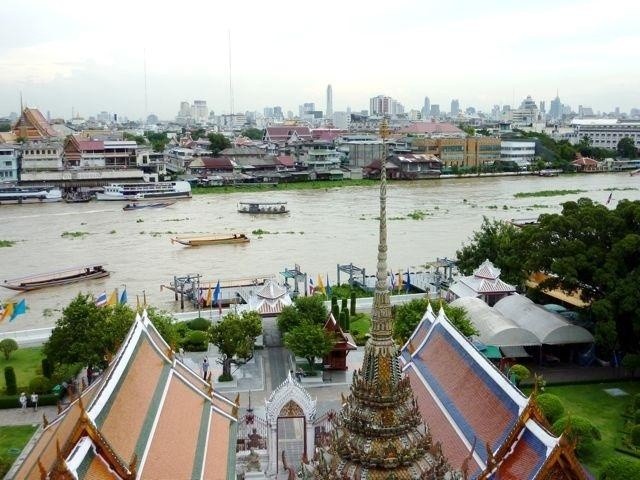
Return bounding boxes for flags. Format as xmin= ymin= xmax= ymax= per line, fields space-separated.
xmin=197 ymin=288 xmax=205 ymax=308
xmin=0 ymin=301 xmax=8 ymax=314
xmin=8 ymin=298 xmax=27 ymax=323
xmin=404 ymin=270 xmax=411 ymax=290
xmin=388 ymin=271 xmax=396 ymax=292
xmin=308 ymin=277 xmax=314 ymax=295
xmin=107 ymin=291 xmax=117 ymax=306
xmin=397 ymin=272 xmax=404 ymax=291
xmin=206 ymin=286 xmax=212 ymax=307
xmin=118 ymin=289 xmax=129 ymax=305
xmin=317 ymin=274 xmax=326 ymax=297
xmin=213 ymin=283 xmax=220 ymax=307
xmin=93 ymin=291 xmax=106 ymax=309
xmin=326 ymin=274 xmax=332 ymax=296
xmin=0 ymin=303 xmax=14 ymax=325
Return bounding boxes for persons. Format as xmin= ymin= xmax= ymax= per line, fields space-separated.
xmin=202 ymin=358 xmax=209 ymax=381
xmin=19 ymin=392 xmax=28 ymax=415
xmin=30 ymin=391 xmax=40 ymax=412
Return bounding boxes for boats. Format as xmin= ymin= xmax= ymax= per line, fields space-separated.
xmin=94 ymin=181 xmax=192 ymax=202
xmin=237 ymin=200 xmax=291 ymax=215
xmin=170 ymin=233 xmax=251 ymax=246
xmin=123 ymin=201 xmax=175 ymax=211
xmin=161 ymin=273 xmax=299 ymax=307
xmin=0 ymin=185 xmax=63 ymax=205
xmin=0 ymin=264 xmax=111 ymax=293
xmin=63 ymin=189 xmax=93 ymax=202
